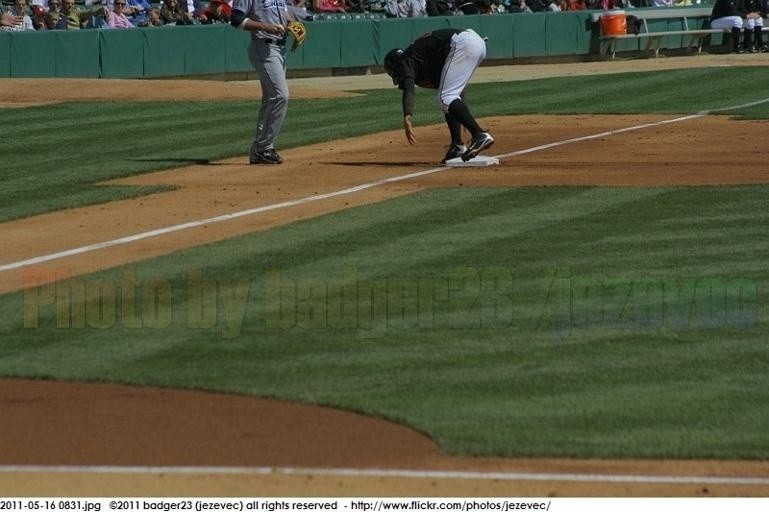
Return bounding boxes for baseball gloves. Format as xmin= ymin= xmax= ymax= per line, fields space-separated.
xmin=286 ymin=20 xmax=307 ymax=52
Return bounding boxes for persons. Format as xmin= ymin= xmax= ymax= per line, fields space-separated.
xmin=230 ymin=0 xmax=309 ymax=167
xmin=748 ymin=0 xmax=769 ymax=54
xmin=708 ymin=0 xmax=763 ymax=54
xmin=382 ymin=27 xmax=498 ymax=165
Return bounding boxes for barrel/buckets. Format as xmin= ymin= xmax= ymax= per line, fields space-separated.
xmin=598 ymin=11 xmax=628 ymax=37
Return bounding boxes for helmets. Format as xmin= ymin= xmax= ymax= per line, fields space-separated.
xmin=382 ymin=48 xmax=406 ymax=75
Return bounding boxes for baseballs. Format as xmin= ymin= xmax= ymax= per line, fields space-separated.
xmin=277 ymin=24 xmax=284 ymax=33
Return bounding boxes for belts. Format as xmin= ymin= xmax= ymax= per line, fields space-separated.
xmin=251 ymin=37 xmax=285 ymax=47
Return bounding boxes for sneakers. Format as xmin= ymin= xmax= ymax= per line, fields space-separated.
xmin=730 ymin=42 xmax=769 ymax=54
xmin=249 ymin=143 xmax=283 ymax=164
xmin=440 ymin=142 xmax=467 ymax=164
xmin=458 ymin=131 xmax=495 ymax=162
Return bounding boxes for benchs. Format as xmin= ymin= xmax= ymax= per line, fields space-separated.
xmin=591 ymin=8 xmax=769 ymax=59
